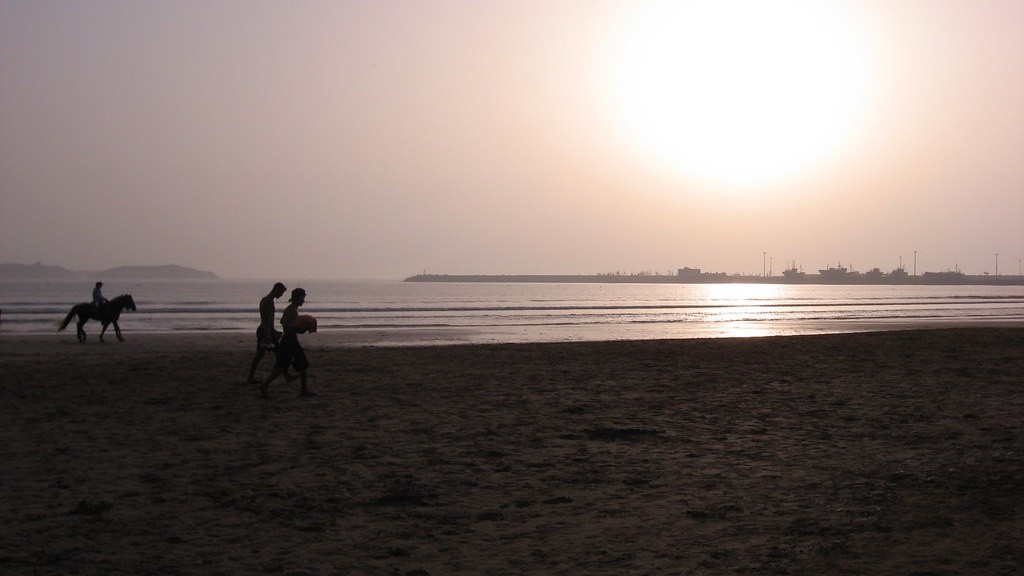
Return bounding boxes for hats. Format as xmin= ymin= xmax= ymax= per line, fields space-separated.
xmin=96 ymin=281 xmax=104 ymax=286
xmin=288 ymin=288 xmax=308 ymax=303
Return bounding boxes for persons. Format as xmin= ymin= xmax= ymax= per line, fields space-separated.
xmin=93 ymin=282 xmax=110 ymax=325
xmin=259 ymin=288 xmax=317 ymax=400
xmin=246 ymin=283 xmax=300 ymax=385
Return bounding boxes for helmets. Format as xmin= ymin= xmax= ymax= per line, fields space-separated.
xmin=290 ymin=315 xmax=317 ymax=333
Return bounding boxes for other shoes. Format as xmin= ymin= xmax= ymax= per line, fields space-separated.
xmin=300 ymin=392 xmax=316 ymax=396
xmin=255 ymin=392 xmax=271 ymax=398
xmin=248 ymin=379 xmax=261 ymax=383
xmin=286 ymin=375 xmax=299 ymax=381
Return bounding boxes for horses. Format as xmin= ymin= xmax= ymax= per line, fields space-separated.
xmin=58 ymin=295 xmax=136 ymax=342
